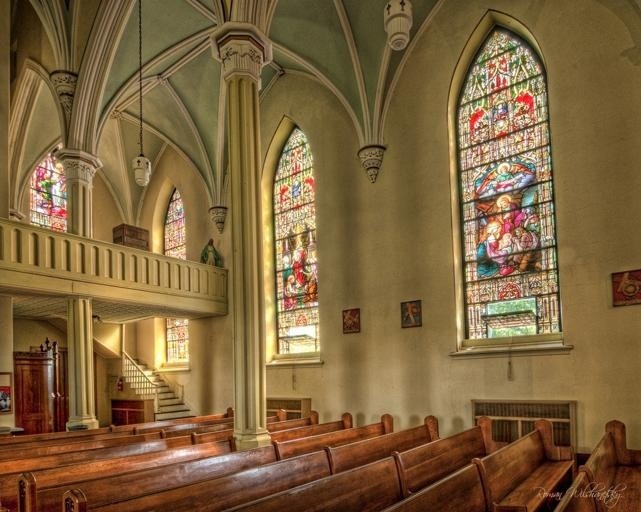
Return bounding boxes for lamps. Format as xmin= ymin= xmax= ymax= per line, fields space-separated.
xmin=382 ymin=0 xmax=415 ymax=51
xmin=132 ymin=0 xmax=152 ymax=186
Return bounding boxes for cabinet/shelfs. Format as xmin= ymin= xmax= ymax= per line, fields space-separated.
xmin=110 ymin=397 xmax=155 ymax=425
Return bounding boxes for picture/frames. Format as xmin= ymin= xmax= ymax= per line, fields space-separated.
xmin=399 ymin=298 xmax=423 ymax=329
xmin=341 ymin=308 xmax=362 ymax=334
xmin=609 ymin=266 xmax=641 ymax=308
xmin=0 ymin=371 xmax=14 ymax=416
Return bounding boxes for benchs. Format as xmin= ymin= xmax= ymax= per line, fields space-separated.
xmin=0 ymin=408 xmax=641 ymax=512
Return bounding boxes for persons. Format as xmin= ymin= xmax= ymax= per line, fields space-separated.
xmin=282 ymin=220 xmax=319 ymax=308
xmin=473 ymin=159 xmax=543 ymax=277
xmin=199 ymin=238 xmax=223 ymax=267
